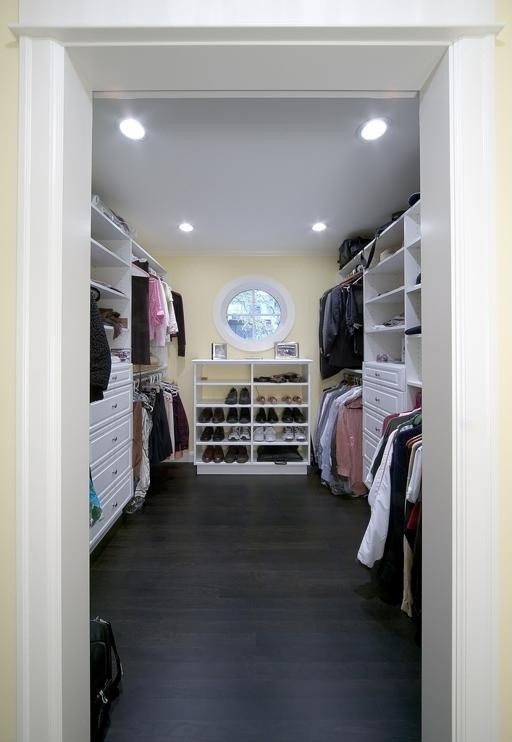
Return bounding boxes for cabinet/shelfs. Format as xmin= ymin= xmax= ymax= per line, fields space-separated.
xmin=337 ymin=199 xmax=421 ymax=507
xmin=91 ymin=205 xmax=168 ymax=550
xmin=191 ymin=358 xmax=311 ymax=477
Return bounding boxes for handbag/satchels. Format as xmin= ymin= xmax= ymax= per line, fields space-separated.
xmin=90 ymin=618 xmax=125 ymax=742
xmin=256 ymin=445 xmax=303 ymax=465
xmin=337 ymin=236 xmax=377 ymax=269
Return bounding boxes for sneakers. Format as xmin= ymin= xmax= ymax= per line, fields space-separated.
xmin=228 ymin=426 xmax=307 ymax=442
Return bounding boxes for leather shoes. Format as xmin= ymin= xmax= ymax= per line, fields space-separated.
xmin=200 ymin=426 xmax=249 ymax=462
xmin=199 ymin=388 xmax=305 ymax=423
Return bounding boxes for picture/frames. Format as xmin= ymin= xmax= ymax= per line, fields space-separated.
xmin=274 ymin=341 xmax=300 ymax=358
xmin=212 ymin=343 xmax=229 ymax=359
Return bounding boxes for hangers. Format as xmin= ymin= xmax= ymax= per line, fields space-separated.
xmin=157 ymin=372 xmax=180 ymax=397
xmin=322 ymin=373 xmax=347 ymax=396
